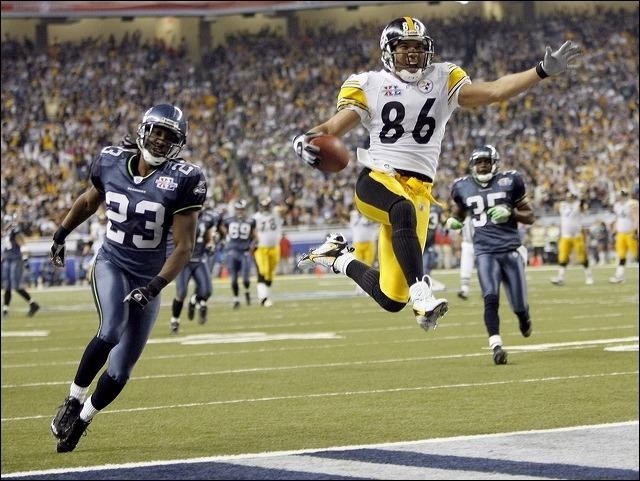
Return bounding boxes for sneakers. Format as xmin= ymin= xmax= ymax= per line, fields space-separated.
xmin=408 ymin=273 xmax=450 ymax=332
xmin=188 ymin=299 xmax=196 ymax=320
xmin=492 ymin=344 xmax=509 ymax=365
xmin=232 ymin=291 xmax=274 ymax=309
xmin=549 ymin=275 xmax=565 ymax=286
xmin=294 ymin=231 xmax=356 ymax=274
xmin=456 ymin=288 xmax=469 ymax=300
xmin=198 ymin=304 xmax=208 ymax=325
xmin=169 ymin=316 xmax=181 ymax=333
xmin=584 ymin=275 xmax=595 ymax=286
xmin=27 ymin=301 xmax=40 ymax=317
xmin=50 ymin=394 xmax=92 ymax=454
xmin=609 ymin=275 xmax=625 ymax=284
xmin=513 ymin=303 xmax=533 ymax=337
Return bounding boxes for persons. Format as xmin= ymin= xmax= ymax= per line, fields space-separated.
xmin=1 ymin=215 xmax=43 ymax=322
xmin=47 ymin=101 xmax=210 ymax=455
xmin=187 ymin=202 xmax=214 ymax=326
xmin=249 ymin=198 xmax=288 ymax=310
xmin=222 ymin=197 xmax=258 ymax=312
xmin=289 ymin=16 xmax=584 ymax=334
xmin=169 ymin=209 xmax=213 ymax=333
xmin=446 ymin=144 xmax=538 ymax=368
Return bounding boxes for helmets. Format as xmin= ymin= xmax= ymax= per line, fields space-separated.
xmin=135 ymin=102 xmax=187 ymax=169
xmin=232 ymin=197 xmax=248 ymax=212
xmin=257 ymin=194 xmax=274 ymax=209
xmin=377 ymin=15 xmax=436 ymax=84
xmin=467 ymin=143 xmax=502 ymax=188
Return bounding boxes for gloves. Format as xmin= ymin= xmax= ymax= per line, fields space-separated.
xmin=535 ymin=39 xmax=583 ymax=80
xmin=446 ymin=216 xmax=464 ymax=230
xmin=486 ymin=203 xmax=516 ymax=221
xmin=47 ymin=224 xmax=71 ymax=269
xmin=291 ymin=130 xmax=325 ymax=170
xmin=122 ymin=275 xmax=169 ymax=310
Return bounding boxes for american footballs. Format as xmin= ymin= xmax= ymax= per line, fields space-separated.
xmin=308 ymin=133 xmax=350 ymax=171
